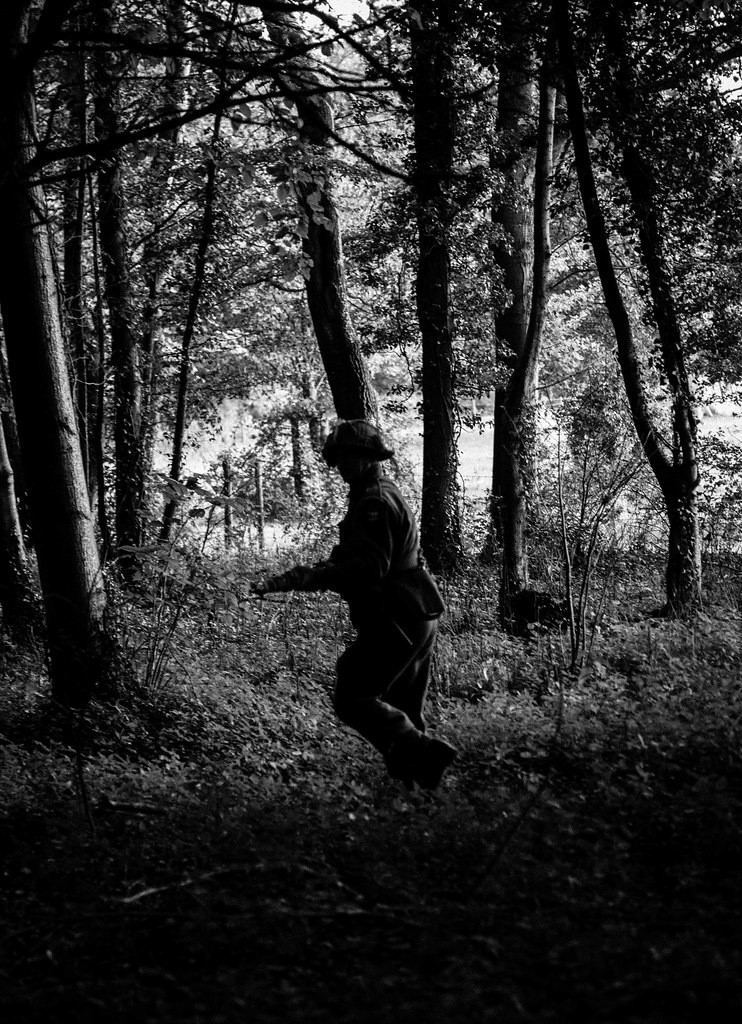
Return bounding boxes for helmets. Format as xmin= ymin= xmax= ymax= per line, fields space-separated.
xmin=320 ymin=419 xmax=395 ymax=467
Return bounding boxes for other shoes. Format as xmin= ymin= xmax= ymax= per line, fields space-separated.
xmin=405 ymin=735 xmax=459 ymax=792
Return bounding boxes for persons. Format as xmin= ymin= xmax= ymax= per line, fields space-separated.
xmin=285 ymin=420 xmax=456 ymax=791
